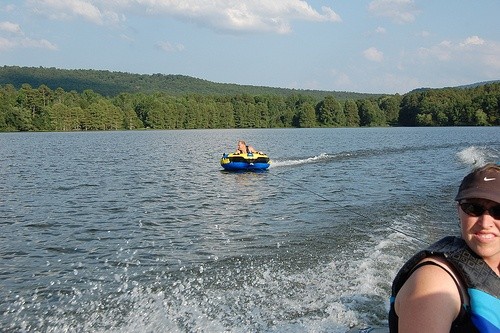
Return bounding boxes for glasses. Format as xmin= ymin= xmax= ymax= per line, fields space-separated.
xmin=457 ymin=200 xmax=500 ymax=220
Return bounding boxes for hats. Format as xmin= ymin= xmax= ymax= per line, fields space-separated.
xmin=454 ymin=164 xmax=500 ymax=203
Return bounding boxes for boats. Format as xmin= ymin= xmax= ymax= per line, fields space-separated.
xmin=221 ymin=152 xmax=271 ymax=171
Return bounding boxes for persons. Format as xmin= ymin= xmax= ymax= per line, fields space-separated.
xmin=233 ymin=140 xmax=256 ymax=153
xmin=388 ymin=163 xmax=500 ymax=333
